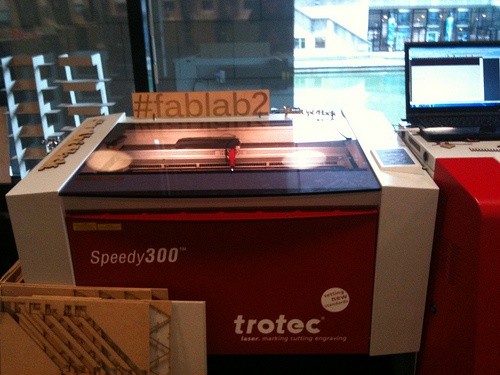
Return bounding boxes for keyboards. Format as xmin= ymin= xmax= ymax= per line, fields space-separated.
xmin=467 ymin=143 xmax=500 ymax=154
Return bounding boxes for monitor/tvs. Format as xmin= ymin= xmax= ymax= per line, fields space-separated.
xmin=405 ymin=40 xmax=500 ymax=123
